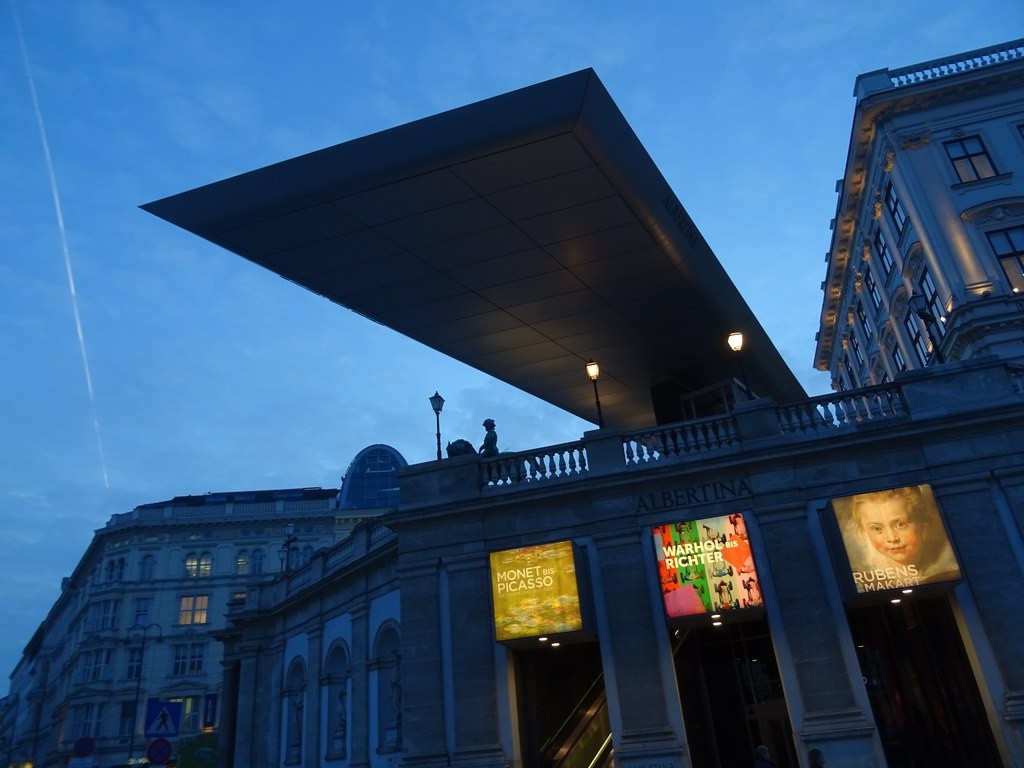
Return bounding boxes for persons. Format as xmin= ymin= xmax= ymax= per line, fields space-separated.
xmin=854 ymin=485 xmax=961 ymax=592
xmin=479 ymin=420 xmax=498 ymax=456
